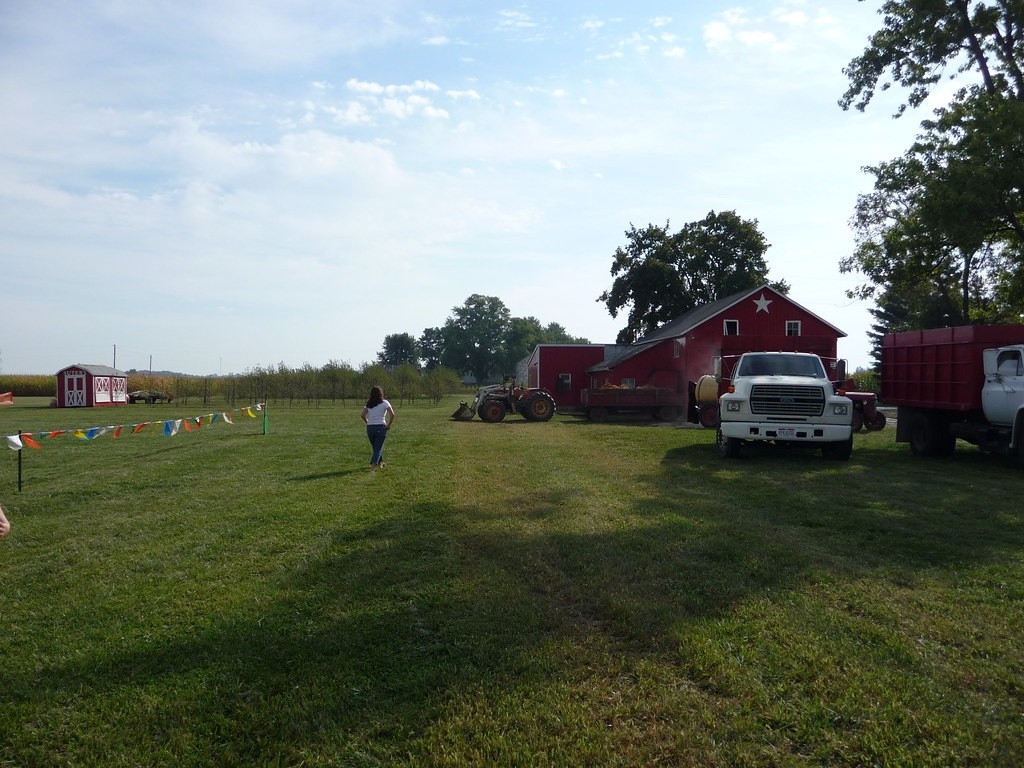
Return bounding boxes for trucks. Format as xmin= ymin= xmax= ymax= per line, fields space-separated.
xmin=716 ymin=334 xmax=855 ymax=462
xmin=880 ymin=322 xmax=1024 ymax=467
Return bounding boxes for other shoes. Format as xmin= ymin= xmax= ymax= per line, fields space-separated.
xmin=378 ymin=461 xmax=385 ymax=468
xmin=370 ymin=463 xmax=376 ymax=470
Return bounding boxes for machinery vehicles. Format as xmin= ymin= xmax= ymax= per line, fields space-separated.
xmin=452 ymin=380 xmax=556 ymax=423
xmin=837 ymin=387 xmax=886 ymax=435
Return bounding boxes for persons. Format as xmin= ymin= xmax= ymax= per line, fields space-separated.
xmin=361 ymin=386 xmax=395 ymax=473
